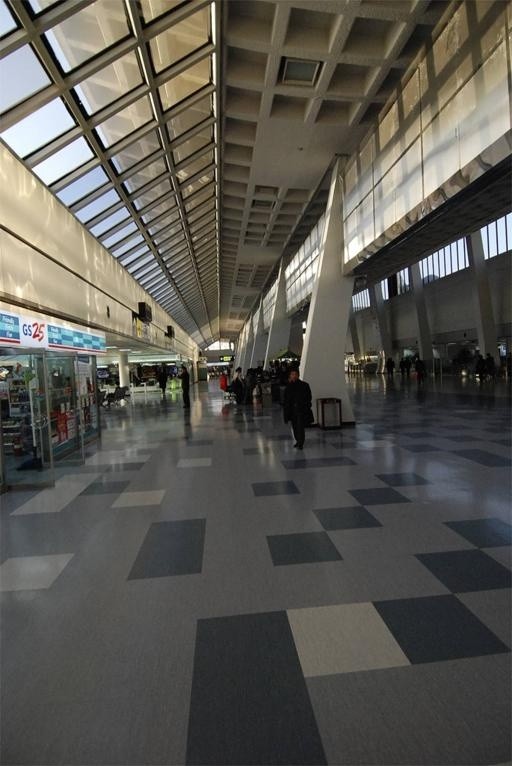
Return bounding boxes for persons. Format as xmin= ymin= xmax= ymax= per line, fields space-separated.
xmin=386 ymin=356 xmax=426 ymax=384
xmin=506 ymin=352 xmax=512 ymax=380
xmin=475 ymin=355 xmax=486 ymax=385
xmin=486 ymin=352 xmax=495 ymax=384
xmin=231 ymin=366 xmax=264 ymax=404
xmin=158 ymin=372 xmax=167 ymax=393
xmin=177 ymin=366 xmax=190 ymax=409
xmin=282 ymin=369 xmax=314 ymax=450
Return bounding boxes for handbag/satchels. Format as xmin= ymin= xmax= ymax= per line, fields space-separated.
xmin=303 ymin=408 xmax=314 ymax=425
xmin=232 ymin=376 xmax=243 ymax=393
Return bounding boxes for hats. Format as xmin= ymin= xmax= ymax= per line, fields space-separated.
xmin=236 ymin=367 xmax=241 ymax=372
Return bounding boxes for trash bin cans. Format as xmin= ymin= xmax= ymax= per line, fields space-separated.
xmin=317 ymin=398 xmax=342 ymax=431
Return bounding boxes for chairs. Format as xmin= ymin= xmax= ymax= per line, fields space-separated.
xmin=220 ymin=374 xmax=236 ymax=400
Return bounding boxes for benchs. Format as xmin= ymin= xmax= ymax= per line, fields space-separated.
xmin=99 ymin=387 xmax=129 ymax=409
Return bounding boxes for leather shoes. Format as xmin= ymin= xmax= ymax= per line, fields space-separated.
xmin=293 ymin=443 xmax=303 ymax=450
xmin=183 ymin=405 xmax=190 ymax=408
